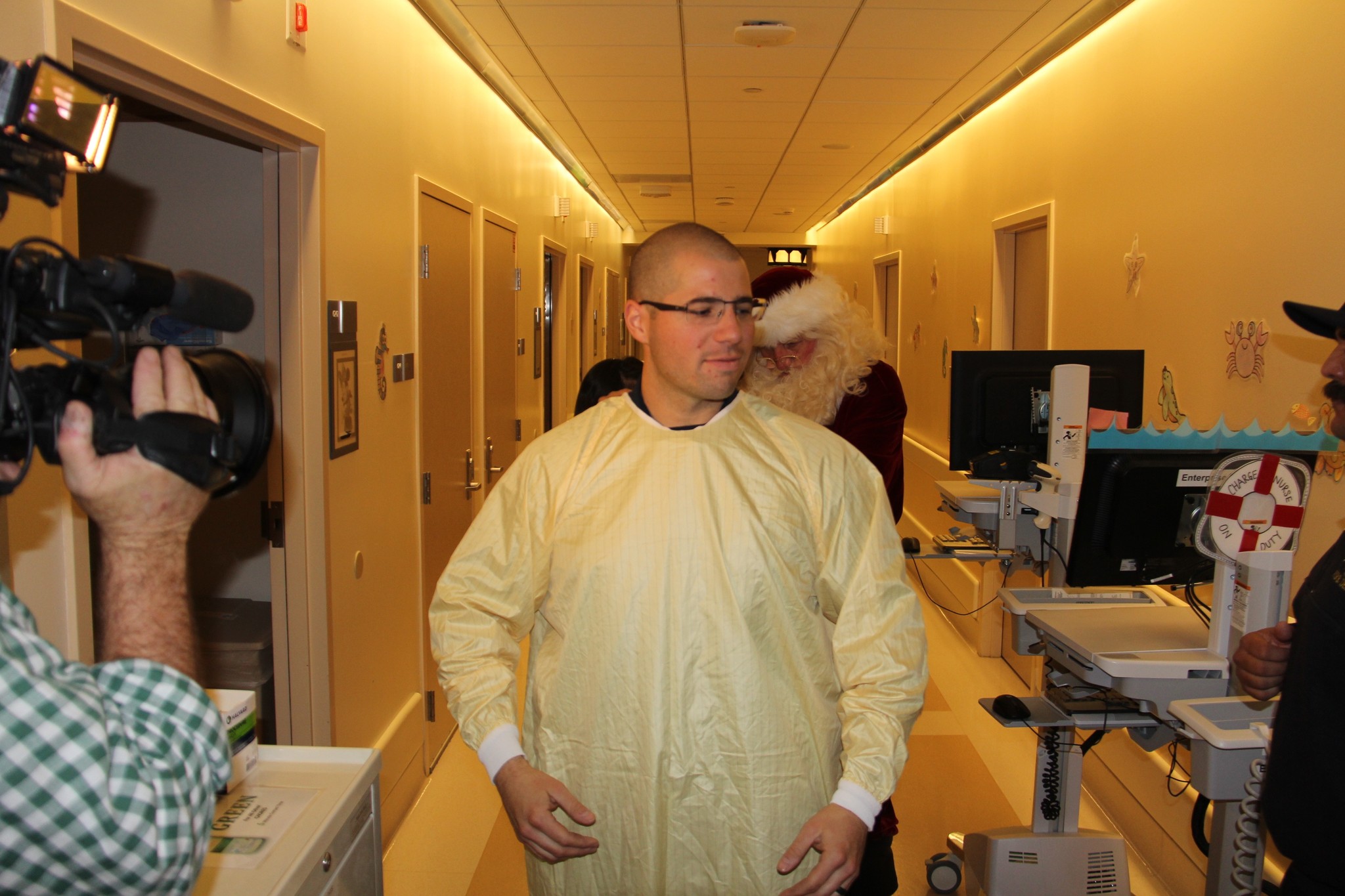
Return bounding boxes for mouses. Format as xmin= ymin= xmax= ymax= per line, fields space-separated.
xmin=993 ymin=695 xmax=1030 ymax=720
xmin=900 ymin=536 xmax=919 ymax=552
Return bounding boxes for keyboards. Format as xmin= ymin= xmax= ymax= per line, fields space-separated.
xmin=932 ymin=532 xmax=990 ymax=551
xmin=1045 ymin=684 xmax=1138 ymax=713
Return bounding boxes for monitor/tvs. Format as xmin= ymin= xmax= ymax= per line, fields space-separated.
xmin=1067 ymin=448 xmax=1317 ymax=587
xmin=949 ymin=348 xmax=1146 ymax=470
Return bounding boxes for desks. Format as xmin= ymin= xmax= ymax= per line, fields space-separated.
xmin=185 ymin=741 xmax=385 ymax=896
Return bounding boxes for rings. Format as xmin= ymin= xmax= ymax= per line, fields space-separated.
xmin=835 ymin=886 xmax=849 ymax=896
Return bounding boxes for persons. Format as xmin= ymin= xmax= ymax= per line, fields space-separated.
xmin=1229 ymin=287 xmax=1345 ymax=896
xmin=750 ymin=264 xmax=904 ymax=895
xmin=427 ymin=222 xmax=929 ymax=896
xmin=1 ymin=345 xmax=233 ymax=894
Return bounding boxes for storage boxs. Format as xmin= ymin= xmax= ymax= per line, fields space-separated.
xmin=203 ymin=687 xmax=260 ymax=797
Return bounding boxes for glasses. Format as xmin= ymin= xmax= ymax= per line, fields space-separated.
xmin=755 ymin=341 xmax=810 ymax=368
xmin=639 ymin=294 xmax=770 ymax=325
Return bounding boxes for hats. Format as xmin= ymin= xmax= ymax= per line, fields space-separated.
xmin=1282 ymin=301 xmax=1345 ymax=340
xmin=750 ymin=266 xmax=851 ymax=348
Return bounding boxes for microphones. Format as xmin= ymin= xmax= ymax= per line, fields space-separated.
xmin=167 ymin=269 xmax=256 ymax=333
